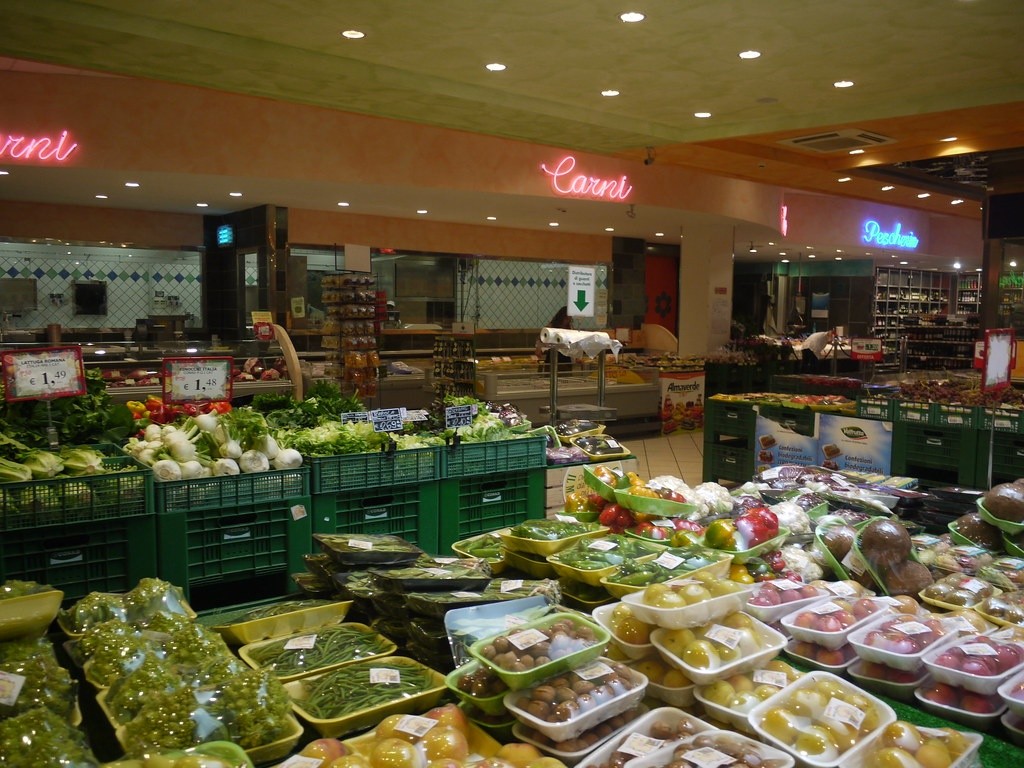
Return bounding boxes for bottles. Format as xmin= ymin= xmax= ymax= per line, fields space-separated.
xmin=958 ymin=279 xmax=982 ymax=314
xmin=904 ymin=329 xmax=979 ymax=370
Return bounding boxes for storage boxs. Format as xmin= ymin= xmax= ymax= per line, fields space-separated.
xmin=702 ymin=376 xmax=1024 ymax=490
xmin=0 ymin=514 xmax=157 ymax=610
xmin=439 ymin=469 xmax=546 ymax=557
xmin=440 ymin=436 xmax=547 ymax=479
xmin=0 ymin=444 xmax=155 ymax=534
xmin=154 ymin=462 xmax=311 ymax=514
xmin=313 ymin=480 xmax=440 ymax=555
xmin=310 ymin=445 xmax=440 ymax=495
xmin=158 ymin=494 xmax=312 ymax=607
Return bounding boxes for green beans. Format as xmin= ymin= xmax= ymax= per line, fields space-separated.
xmin=219 ymin=533 xmax=559 ymax=719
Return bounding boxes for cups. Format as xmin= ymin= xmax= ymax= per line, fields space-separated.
xmin=47 ymin=324 xmax=61 ymax=342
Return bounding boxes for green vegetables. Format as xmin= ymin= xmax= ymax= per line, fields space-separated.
xmin=253 ymin=379 xmax=513 ymax=457
xmin=0 ymin=367 xmax=145 ymax=513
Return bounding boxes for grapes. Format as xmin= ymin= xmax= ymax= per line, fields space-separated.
xmin=877 ymin=378 xmax=1024 ymax=410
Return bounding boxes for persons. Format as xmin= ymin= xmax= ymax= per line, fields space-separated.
xmin=388 ymin=301 xmax=401 ymax=325
xmin=801 ymin=331 xmax=834 ymax=374
xmin=787 ymin=307 xmax=810 ymax=339
xmin=535 ymin=306 xmax=574 ymax=378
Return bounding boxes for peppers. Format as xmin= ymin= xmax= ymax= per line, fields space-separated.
xmin=126 ymin=396 xmax=231 ymax=438
xmin=456 ymin=518 xmax=724 ymax=601
xmin=565 ymin=466 xmax=803 ymax=584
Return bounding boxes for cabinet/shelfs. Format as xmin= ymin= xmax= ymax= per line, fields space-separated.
xmin=788 ymin=260 xmax=1024 ymax=372
